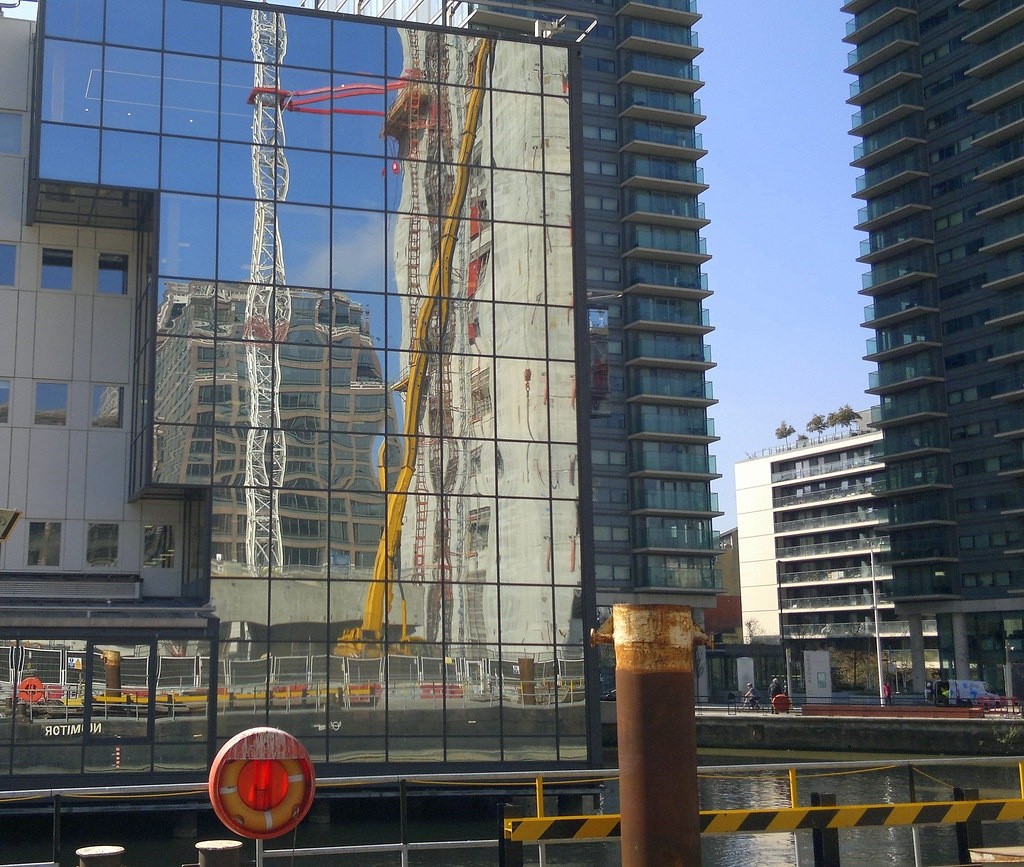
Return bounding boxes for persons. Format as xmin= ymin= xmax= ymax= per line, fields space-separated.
xmin=768 ymin=678 xmax=782 ymax=714
xmin=744 ymin=683 xmax=760 ymax=707
xmin=879 ymin=682 xmax=894 ymax=706
xmin=942 ymin=690 xmax=949 ymax=707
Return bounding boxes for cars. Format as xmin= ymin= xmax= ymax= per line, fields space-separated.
xmin=601 ymin=687 xmax=616 ymax=701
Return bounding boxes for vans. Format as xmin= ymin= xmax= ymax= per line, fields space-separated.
xmin=925 ymin=678 xmax=1002 ymax=709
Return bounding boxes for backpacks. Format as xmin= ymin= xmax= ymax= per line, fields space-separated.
xmin=771 ymin=684 xmax=778 ymax=695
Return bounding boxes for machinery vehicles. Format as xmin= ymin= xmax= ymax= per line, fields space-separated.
xmin=330 ymin=37 xmax=501 ymax=664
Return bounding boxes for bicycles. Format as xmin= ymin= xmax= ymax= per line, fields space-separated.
xmin=738 ymin=695 xmax=770 ymax=713
